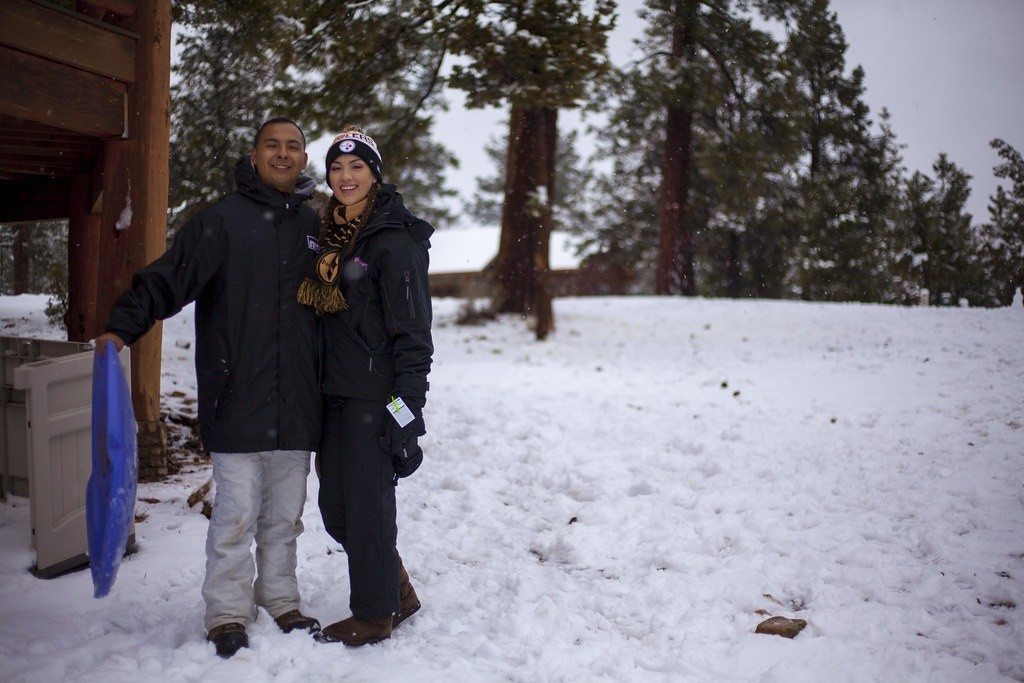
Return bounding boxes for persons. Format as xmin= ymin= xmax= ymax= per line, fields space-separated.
xmin=95 ymin=118 xmax=330 ymax=658
xmin=316 ymin=131 xmax=437 ymax=649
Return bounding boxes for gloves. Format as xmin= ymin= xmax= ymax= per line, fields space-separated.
xmin=391 ymin=433 xmax=424 ymax=479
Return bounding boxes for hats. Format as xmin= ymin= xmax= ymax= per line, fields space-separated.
xmin=324 ymin=126 xmax=383 ymax=190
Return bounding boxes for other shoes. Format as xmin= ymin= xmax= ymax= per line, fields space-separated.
xmin=391 ymin=562 xmax=422 ymax=629
xmin=321 ymin=613 xmax=393 ymax=646
xmin=206 ymin=622 xmax=249 ymax=656
xmin=275 ymin=610 xmax=322 ymax=637
